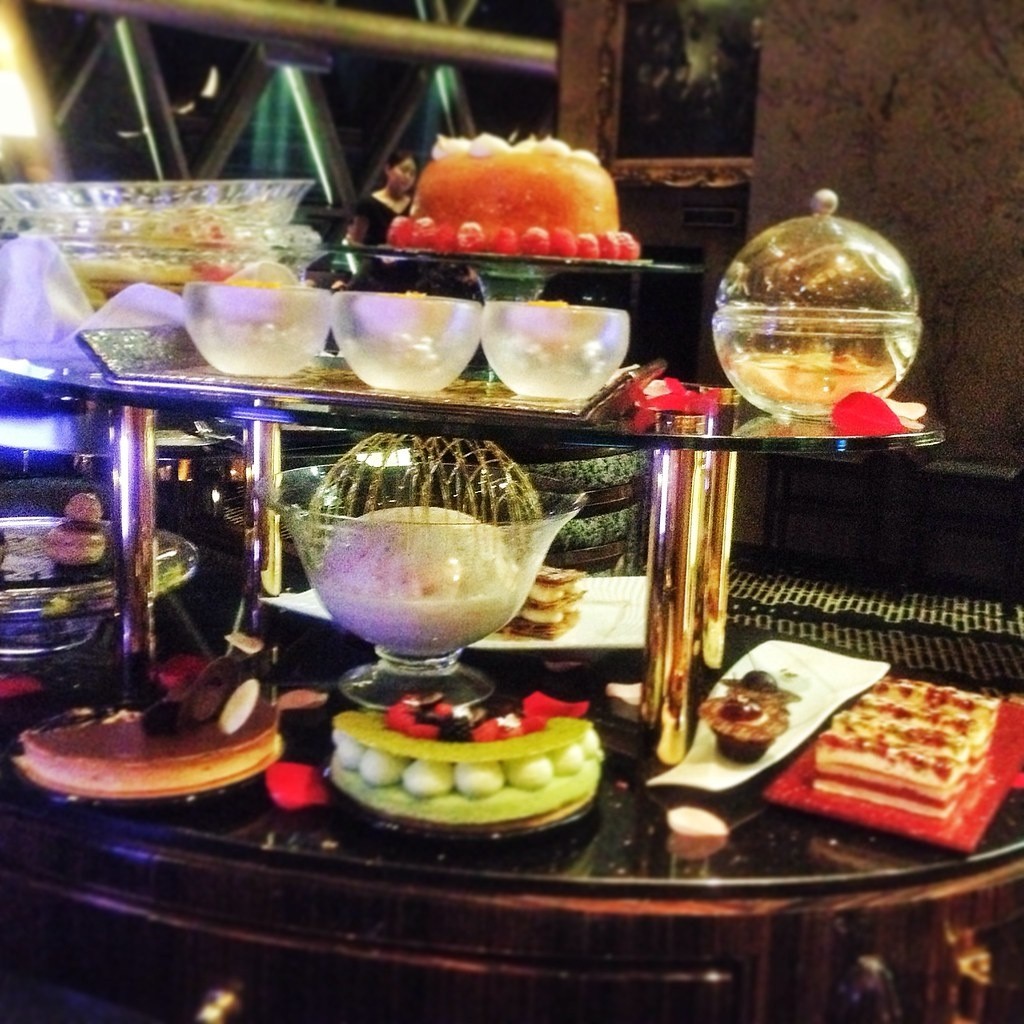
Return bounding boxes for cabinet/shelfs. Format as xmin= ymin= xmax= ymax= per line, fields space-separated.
xmin=0 ymin=241 xmax=1024 ymax=1024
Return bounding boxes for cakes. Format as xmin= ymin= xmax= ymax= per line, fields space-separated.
xmin=389 ymin=126 xmax=639 ymax=261
xmin=328 ymin=674 xmax=603 ymax=826
xmin=13 ymin=646 xmax=282 ymax=791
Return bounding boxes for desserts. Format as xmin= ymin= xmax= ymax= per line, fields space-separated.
xmin=695 ymin=666 xmax=799 ymax=762
xmin=813 ymin=674 xmax=1004 ymax=821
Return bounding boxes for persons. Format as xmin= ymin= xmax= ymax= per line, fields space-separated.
xmin=340 ymin=146 xmax=422 ymax=292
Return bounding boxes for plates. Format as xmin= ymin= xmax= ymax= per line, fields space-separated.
xmin=260 ymin=573 xmax=648 ymax=651
xmin=763 ymin=700 xmax=1024 ymax=855
xmin=645 ymin=638 xmax=891 ymax=792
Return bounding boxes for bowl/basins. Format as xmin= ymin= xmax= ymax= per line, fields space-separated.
xmin=330 ymin=290 xmax=483 ymax=392
xmin=1 ymin=173 xmax=328 ymax=291
xmin=253 ymin=465 xmax=590 ymax=711
xmin=181 ymin=281 xmax=333 ymax=379
xmin=480 ymin=301 xmax=629 ymax=400
